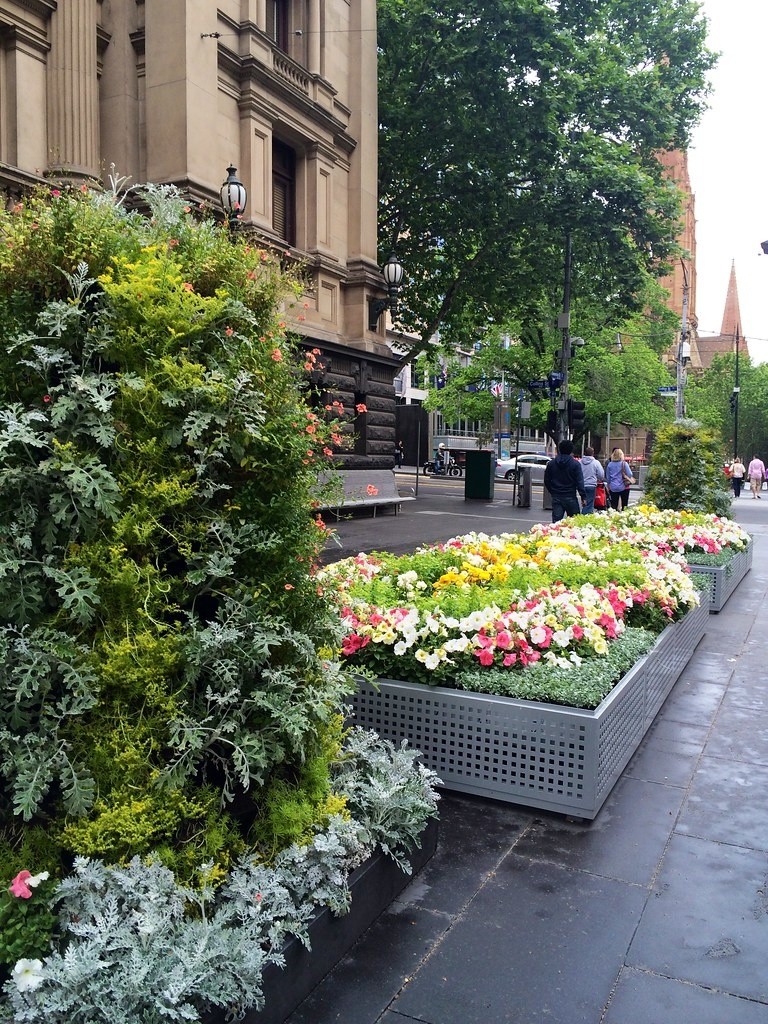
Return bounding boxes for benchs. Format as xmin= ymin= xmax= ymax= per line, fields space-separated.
xmin=300 ymin=469 xmax=416 ymax=522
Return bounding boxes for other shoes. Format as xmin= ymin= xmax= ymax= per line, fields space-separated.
xmin=757 ymin=496 xmax=760 ymax=498
xmin=752 ymin=497 xmax=756 ymax=499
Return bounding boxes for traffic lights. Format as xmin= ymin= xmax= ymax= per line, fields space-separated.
xmin=569 ymin=398 xmax=586 ymax=434
xmin=600 ymin=412 xmax=608 ymax=422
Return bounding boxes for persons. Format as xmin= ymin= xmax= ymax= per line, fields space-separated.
xmin=747 ymin=455 xmax=766 ymax=499
xmin=578 ymin=447 xmax=604 ymax=514
xmin=604 ymin=448 xmax=633 ymax=511
xmin=544 ymin=440 xmax=587 ymax=523
xmin=729 ymin=458 xmax=745 ymax=498
xmin=395 ymin=441 xmax=404 ymax=468
xmin=435 ymin=443 xmax=452 ymax=469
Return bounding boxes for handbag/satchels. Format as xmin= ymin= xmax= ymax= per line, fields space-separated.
xmin=744 ymin=482 xmax=750 ymax=490
xmin=729 ymin=466 xmax=734 ymax=477
xmin=622 ymin=461 xmax=636 ymax=485
xmin=594 ymin=486 xmax=605 ymax=508
xmin=762 ymin=482 xmax=767 ymax=491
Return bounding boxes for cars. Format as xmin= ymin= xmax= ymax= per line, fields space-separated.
xmin=495 ymin=454 xmax=552 ymax=484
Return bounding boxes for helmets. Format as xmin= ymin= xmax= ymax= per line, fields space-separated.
xmin=439 ymin=443 xmax=445 ymax=449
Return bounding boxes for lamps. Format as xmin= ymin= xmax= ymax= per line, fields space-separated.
xmin=367 ymin=248 xmax=403 ymax=333
xmin=220 ymin=163 xmax=247 ymax=245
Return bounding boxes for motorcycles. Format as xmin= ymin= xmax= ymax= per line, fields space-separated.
xmin=422 ymin=455 xmax=462 ymax=477
